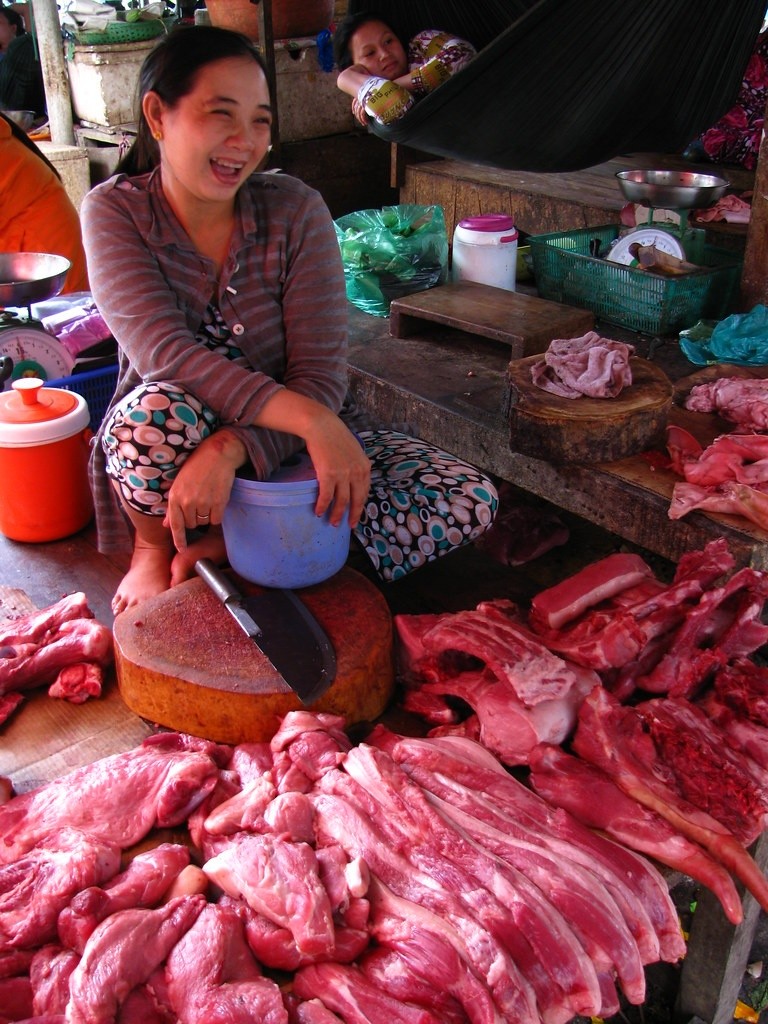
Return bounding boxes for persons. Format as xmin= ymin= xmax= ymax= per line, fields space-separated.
xmin=78 ymin=26 xmax=499 ymax=614
xmin=0 ymin=7 xmax=47 ymax=131
xmin=0 ymin=112 xmax=90 ymax=295
xmin=333 ymin=10 xmax=477 ymax=125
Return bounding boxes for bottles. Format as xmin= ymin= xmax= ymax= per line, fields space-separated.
xmin=451 ymin=213 xmax=519 ymax=292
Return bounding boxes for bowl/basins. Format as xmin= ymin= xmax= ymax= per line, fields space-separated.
xmin=0 ymin=109 xmax=36 ymax=131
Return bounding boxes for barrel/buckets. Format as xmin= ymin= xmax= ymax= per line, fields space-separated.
xmin=220 ymin=479 xmax=351 ymax=589
xmin=0 ymin=378 xmax=92 ymax=543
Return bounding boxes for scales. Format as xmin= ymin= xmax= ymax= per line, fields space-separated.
xmin=599 ymin=169 xmax=731 ymax=329
xmin=0 ymin=253 xmax=80 ymax=397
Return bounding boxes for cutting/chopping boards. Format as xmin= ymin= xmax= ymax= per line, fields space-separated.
xmin=112 ymin=565 xmax=393 ymax=744
xmin=501 ymin=353 xmax=675 ymax=462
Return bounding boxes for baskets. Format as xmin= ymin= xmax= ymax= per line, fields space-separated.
xmin=526 ymin=223 xmax=744 ymax=338
xmin=45 ymin=366 xmax=120 ymax=434
xmin=70 ymin=14 xmax=178 ymax=45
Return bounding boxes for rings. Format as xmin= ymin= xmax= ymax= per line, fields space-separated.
xmin=196 ymin=514 xmax=208 ymax=519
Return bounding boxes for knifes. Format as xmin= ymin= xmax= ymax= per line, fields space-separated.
xmin=194 ymin=557 xmax=336 ymax=705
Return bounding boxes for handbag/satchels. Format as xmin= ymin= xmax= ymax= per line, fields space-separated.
xmin=333 ymin=204 xmax=449 ymax=317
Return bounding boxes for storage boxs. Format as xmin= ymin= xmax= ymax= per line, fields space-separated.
xmin=63 ymin=38 xmax=165 ymax=128
xmin=526 ymin=223 xmax=741 ymax=340
xmin=252 ymin=37 xmax=357 ymax=143
